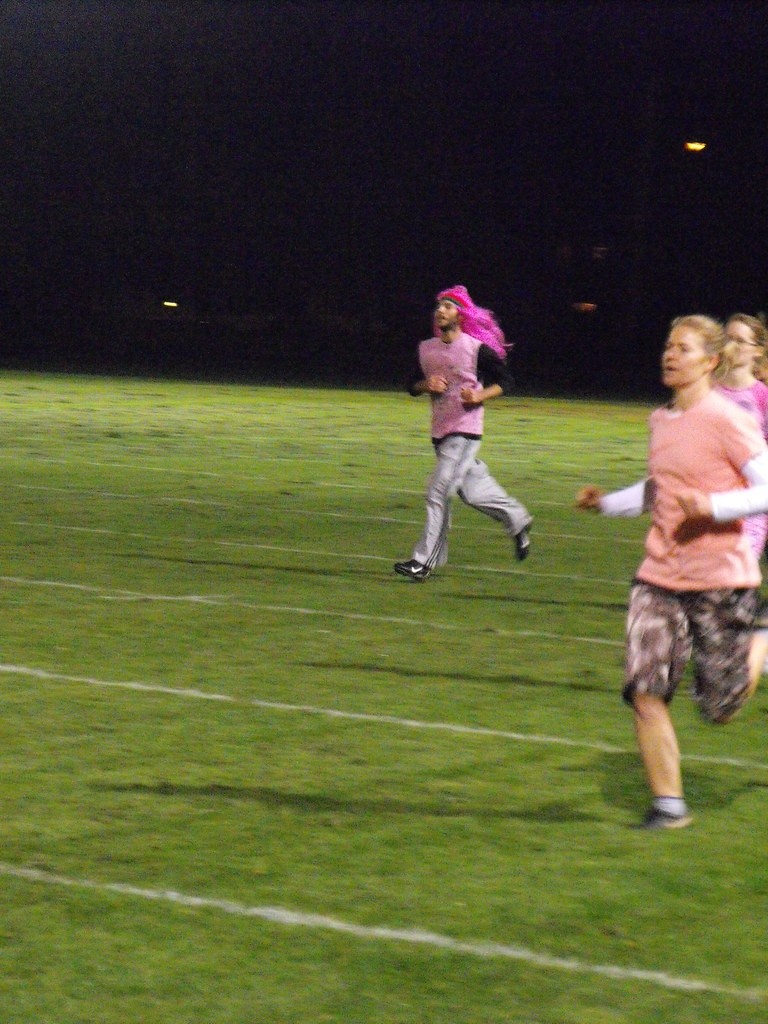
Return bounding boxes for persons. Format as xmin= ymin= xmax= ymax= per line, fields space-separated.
xmin=573 ymin=316 xmax=768 ymax=828
xmin=394 ymin=284 xmax=535 ymax=581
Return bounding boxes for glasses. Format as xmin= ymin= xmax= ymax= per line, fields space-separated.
xmin=725 ymin=334 xmax=757 ymax=347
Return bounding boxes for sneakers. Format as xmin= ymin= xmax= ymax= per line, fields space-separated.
xmin=514 ymin=518 xmax=533 ymax=561
xmin=630 ymin=808 xmax=693 ymax=831
xmin=395 ymin=559 xmax=431 ymax=584
